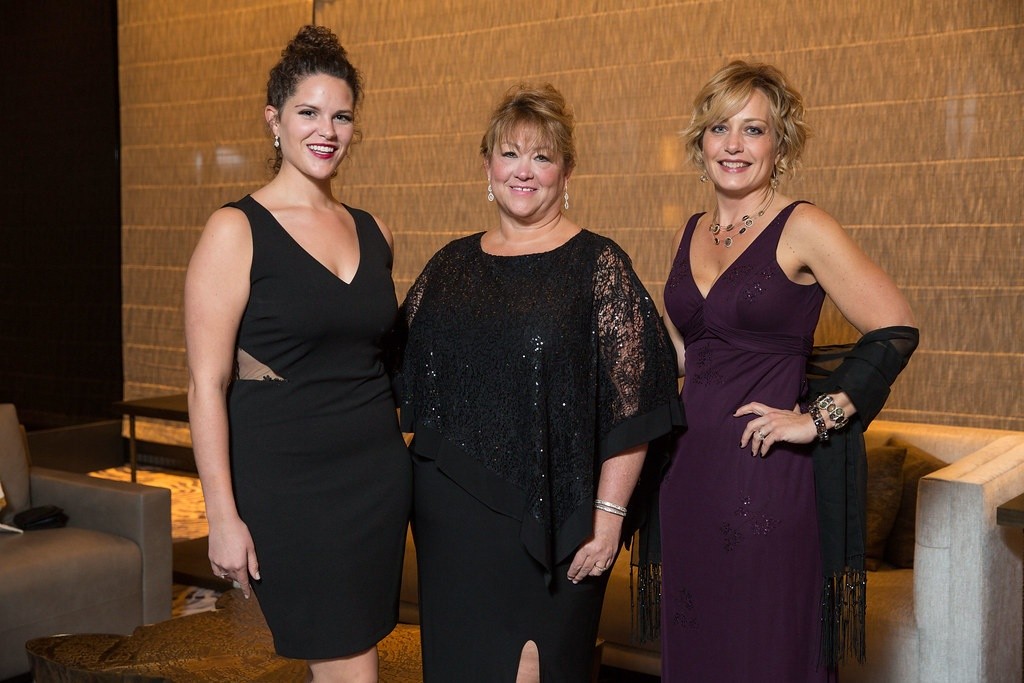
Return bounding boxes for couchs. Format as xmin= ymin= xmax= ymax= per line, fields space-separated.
xmin=0 ymin=404 xmax=172 ymax=683
xmin=393 ymin=418 xmax=1024 ymax=683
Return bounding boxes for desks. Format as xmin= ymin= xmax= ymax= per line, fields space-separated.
xmin=111 ymin=393 xmax=233 ymax=593
xmin=25 ymin=587 xmax=604 ymax=683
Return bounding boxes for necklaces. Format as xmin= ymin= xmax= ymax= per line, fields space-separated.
xmin=709 ymin=188 xmax=775 ymax=247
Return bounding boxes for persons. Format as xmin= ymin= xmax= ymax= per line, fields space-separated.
xmin=398 ymin=82 xmax=688 ymax=683
xmin=184 ymin=25 xmax=411 ymax=683
xmin=630 ymin=60 xmax=919 ymax=683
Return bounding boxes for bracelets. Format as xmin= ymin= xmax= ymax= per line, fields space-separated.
xmin=815 ymin=394 xmax=848 ymax=430
xmin=596 ymin=499 xmax=627 ymax=516
xmin=807 ymin=402 xmax=829 ymax=441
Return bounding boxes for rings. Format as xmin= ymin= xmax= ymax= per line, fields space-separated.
xmin=594 ymin=565 xmax=605 ymax=572
xmin=220 ymin=573 xmax=228 ymax=576
xmin=757 ymin=429 xmax=765 ymax=439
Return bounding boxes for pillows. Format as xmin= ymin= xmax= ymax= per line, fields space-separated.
xmin=866 ymin=437 xmax=907 ymax=572
xmin=886 ymin=459 xmax=934 ymax=569
